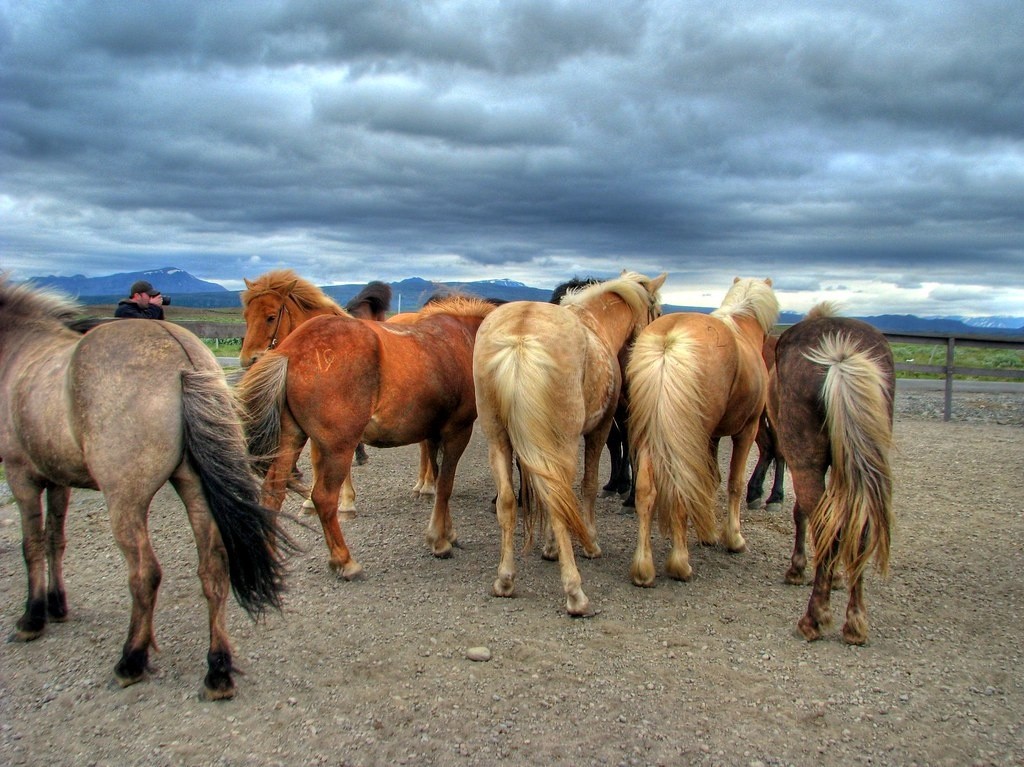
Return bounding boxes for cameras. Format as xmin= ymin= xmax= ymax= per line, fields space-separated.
xmin=153 ymin=295 xmax=171 ymax=305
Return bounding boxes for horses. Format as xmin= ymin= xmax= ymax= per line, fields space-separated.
xmin=0 ymin=275 xmax=314 ymax=702
xmin=234 ymin=267 xmax=897 ymax=649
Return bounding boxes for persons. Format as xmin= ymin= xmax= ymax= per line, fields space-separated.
xmin=113 ymin=280 xmax=172 ymax=321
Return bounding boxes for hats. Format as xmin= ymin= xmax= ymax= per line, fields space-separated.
xmin=131 ymin=280 xmax=161 ymax=296
